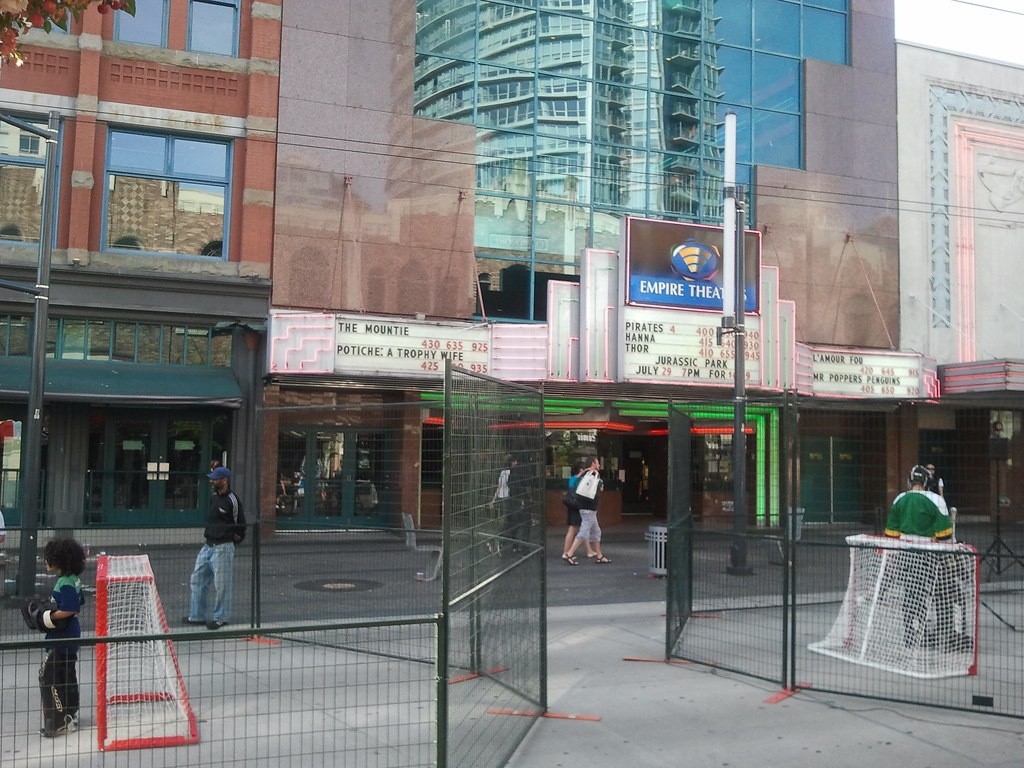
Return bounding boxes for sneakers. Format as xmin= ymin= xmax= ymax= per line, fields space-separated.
xmin=206 ymin=621 xmax=226 ymax=629
xmin=183 ymin=617 xmax=205 ymax=624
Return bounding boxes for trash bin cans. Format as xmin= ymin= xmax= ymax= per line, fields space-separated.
xmin=643 ymin=522 xmax=668 ymax=575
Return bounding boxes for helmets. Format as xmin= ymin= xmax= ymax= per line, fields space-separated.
xmin=908 ymin=465 xmax=932 ymax=490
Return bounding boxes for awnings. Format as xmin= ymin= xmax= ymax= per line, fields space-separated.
xmin=0 ymin=353 xmax=243 ymax=408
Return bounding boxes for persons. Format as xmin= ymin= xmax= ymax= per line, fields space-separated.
xmin=562 ymin=456 xmax=613 ymax=565
xmin=884 ymin=462 xmax=974 ymax=653
xmin=182 ymin=467 xmax=247 ymax=630
xmin=294 ymin=472 xmax=304 ymax=509
xmin=485 ymin=453 xmax=537 ymax=559
xmin=20 ymin=537 xmax=86 ymax=735
xmin=0 ymin=510 xmax=7 ymax=543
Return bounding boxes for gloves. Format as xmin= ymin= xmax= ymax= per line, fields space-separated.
xmin=22 ymin=601 xmax=56 ymax=630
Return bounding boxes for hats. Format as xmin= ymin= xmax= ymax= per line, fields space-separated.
xmin=206 ymin=467 xmax=231 ymax=480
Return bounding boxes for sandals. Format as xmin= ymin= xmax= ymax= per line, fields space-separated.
xmin=595 ymin=556 xmax=612 ymax=564
xmin=563 ymin=554 xmax=578 ymax=565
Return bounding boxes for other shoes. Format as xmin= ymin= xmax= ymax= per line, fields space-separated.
xmin=38 ymin=715 xmax=79 ymax=737
xmin=512 ymin=547 xmax=521 ymax=553
xmin=496 ymin=541 xmax=503 ymax=556
xmin=487 ymin=542 xmax=495 ymax=554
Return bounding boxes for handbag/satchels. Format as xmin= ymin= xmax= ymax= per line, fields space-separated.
xmin=565 ymin=478 xmax=581 ymax=510
xmin=576 ymin=470 xmax=600 ymax=499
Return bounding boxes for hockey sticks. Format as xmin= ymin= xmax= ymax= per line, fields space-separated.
xmin=951 ymin=506 xmax=958 ymax=544
xmin=964 ymin=587 xmax=1024 ymax=632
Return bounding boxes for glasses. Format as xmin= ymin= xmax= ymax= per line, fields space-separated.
xmin=927 ymin=468 xmax=935 ymax=470
